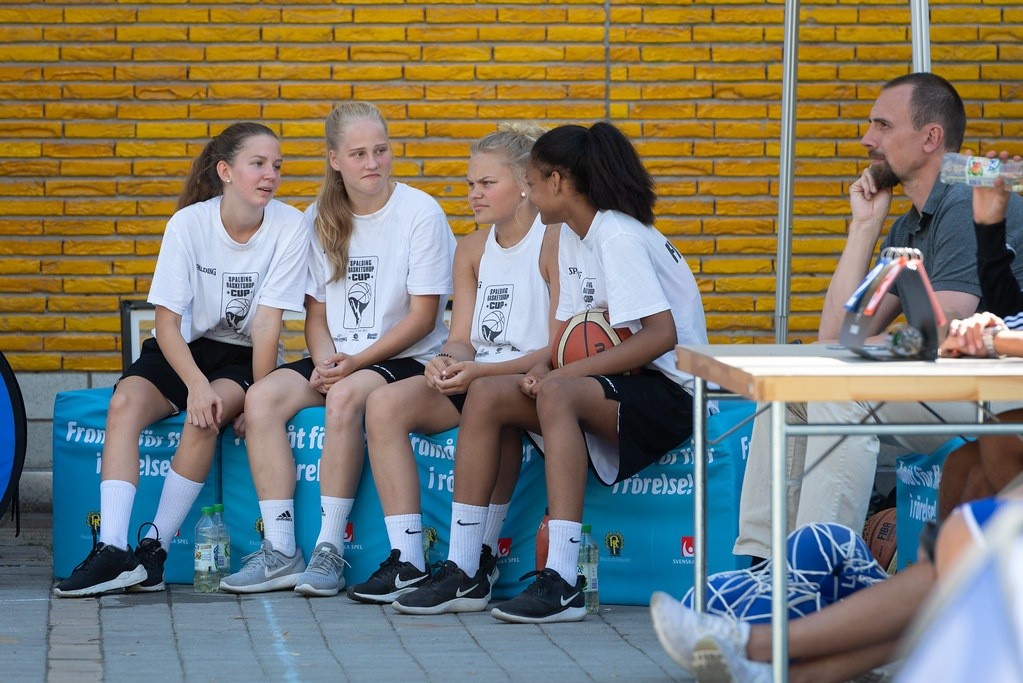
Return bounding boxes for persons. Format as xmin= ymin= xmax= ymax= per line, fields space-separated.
xmin=348 ymin=120 xmax=562 ymax=606
xmin=51 ymin=121 xmax=309 ymax=597
xmin=393 ymin=119 xmax=711 ymax=626
xmin=214 ymin=102 xmax=457 ymax=595
xmin=733 ymin=73 xmax=1023 ymax=561
xmin=651 ymin=148 xmax=1023 ymax=682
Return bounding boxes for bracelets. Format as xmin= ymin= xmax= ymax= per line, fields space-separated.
xmin=436 ymin=353 xmax=451 ymax=358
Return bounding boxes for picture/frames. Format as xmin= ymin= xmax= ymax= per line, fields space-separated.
xmin=120 ymin=299 xmax=156 ymax=377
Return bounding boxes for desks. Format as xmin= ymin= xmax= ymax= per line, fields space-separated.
xmin=674 ymin=339 xmax=1023 ymax=683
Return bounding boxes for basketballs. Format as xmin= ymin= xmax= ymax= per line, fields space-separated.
xmin=861 ymin=506 xmax=902 ymax=578
xmin=551 ymin=310 xmax=642 ymax=379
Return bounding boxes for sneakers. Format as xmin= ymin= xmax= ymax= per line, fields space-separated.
xmin=54 ymin=541 xmax=148 ymax=597
xmin=480 ymin=543 xmax=500 ymax=587
xmin=649 ymin=590 xmax=752 ymax=676
xmin=220 ymin=540 xmax=306 ymax=592
xmin=490 ymin=568 xmax=586 ymax=623
xmin=345 ymin=550 xmax=432 ymax=602
xmin=125 ymin=522 xmax=168 ymax=591
xmin=391 ymin=560 xmax=489 ymax=614
xmin=294 ymin=542 xmax=346 ymax=596
xmin=691 ymin=635 xmax=772 ymax=683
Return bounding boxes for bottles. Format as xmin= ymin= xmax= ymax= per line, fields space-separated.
xmin=885 ymin=325 xmax=922 ymax=355
xmin=578 ymin=525 xmax=598 ymax=615
xmin=535 ymin=507 xmax=551 ymax=575
xmin=212 ymin=503 xmax=230 ymax=588
xmin=940 ymin=152 xmax=1023 ymax=186
xmin=194 ymin=507 xmax=218 ymax=593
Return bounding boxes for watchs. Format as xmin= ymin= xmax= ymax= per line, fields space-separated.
xmin=982 ymin=324 xmax=1009 ymax=359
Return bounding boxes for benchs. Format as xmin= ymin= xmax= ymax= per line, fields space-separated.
xmin=50 ymin=384 xmax=758 ymax=609
